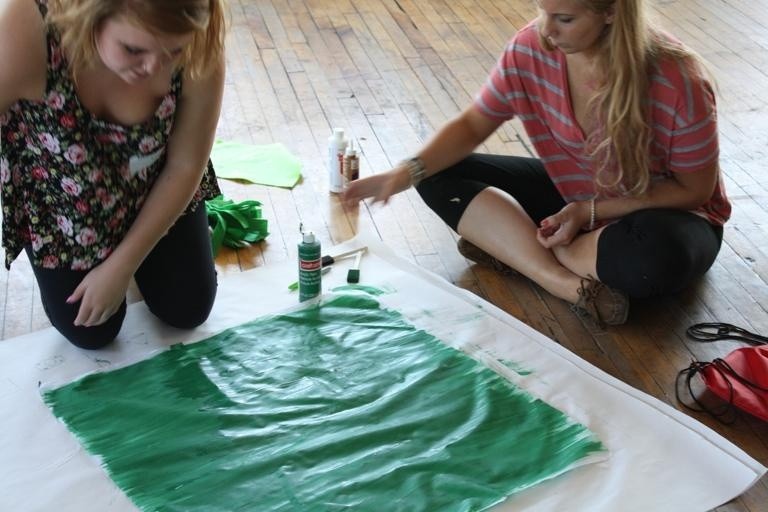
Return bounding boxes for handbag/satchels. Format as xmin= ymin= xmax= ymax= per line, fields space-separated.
xmin=699 ymin=344 xmax=768 ymax=422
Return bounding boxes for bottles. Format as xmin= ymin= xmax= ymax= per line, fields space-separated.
xmin=327 ymin=127 xmax=349 ymax=194
xmin=297 ymin=230 xmax=322 ymax=302
xmin=343 ymin=137 xmax=360 ymax=189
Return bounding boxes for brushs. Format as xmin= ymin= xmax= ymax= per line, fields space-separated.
xmin=321 ymin=247 xmax=367 ymax=283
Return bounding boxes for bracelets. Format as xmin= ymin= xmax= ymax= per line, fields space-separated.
xmin=589 ymin=198 xmax=596 ymax=231
xmin=398 ymin=157 xmax=427 ymax=188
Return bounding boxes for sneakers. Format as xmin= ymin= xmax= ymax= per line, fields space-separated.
xmin=458 ymin=238 xmax=520 ymax=275
xmin=562 ymin=274 xmax=629 ymax=330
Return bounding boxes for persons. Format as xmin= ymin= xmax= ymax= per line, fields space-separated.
xmin=0 ymin=0 xmax=227 ymax=351
xmin=343 ymin=0 xmax=732 ymax=326
